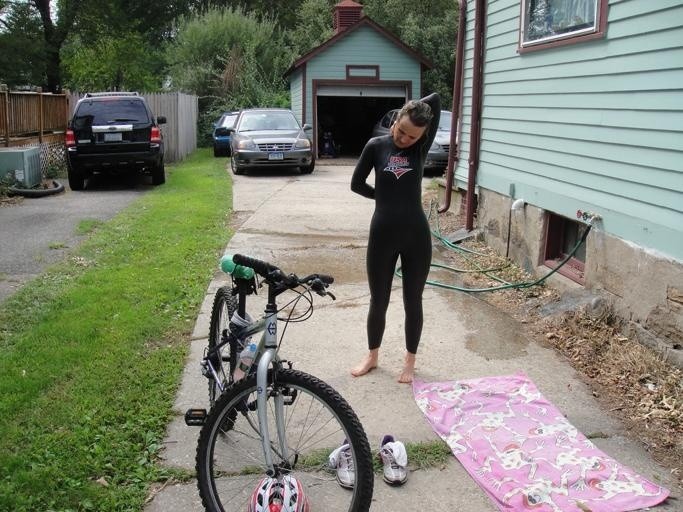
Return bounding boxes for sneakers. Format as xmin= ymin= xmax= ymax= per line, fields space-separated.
xmin=379 ymin=434 xmax=408 ymax=486
xmin=333 ymin=437 xmax=356 ymax=489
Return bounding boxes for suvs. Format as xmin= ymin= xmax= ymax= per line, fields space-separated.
xmin=54 ymin=90 xmax=169 ymax=189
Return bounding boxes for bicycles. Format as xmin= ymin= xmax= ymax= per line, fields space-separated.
xmin=185 ymin=250 xmax=377 ymax=511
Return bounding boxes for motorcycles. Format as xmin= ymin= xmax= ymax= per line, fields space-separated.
xmin=322 ymin=127 xmax=346 ymax=157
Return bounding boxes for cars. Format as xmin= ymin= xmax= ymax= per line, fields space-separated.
xmin=372 ymin=106 xmax=460 ymax=174
xmin=209 ymin=112 xmax=239 ymax=157
xmin=221 ymin=107 xmax=317 ymax=175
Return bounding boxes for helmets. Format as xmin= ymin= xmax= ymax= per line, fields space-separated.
xmin=247 ymin=473 xmax=310 ymax=512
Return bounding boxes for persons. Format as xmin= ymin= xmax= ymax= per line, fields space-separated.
xmin=348 ymin=92 xmax=442 ymax=384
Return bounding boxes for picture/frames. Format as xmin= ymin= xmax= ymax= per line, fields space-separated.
xmin=518 ymin=0 xmax=609 ymax=54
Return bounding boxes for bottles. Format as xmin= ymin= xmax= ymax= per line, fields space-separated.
xmin=232 ymin=343 xmax=257 ymax=384
